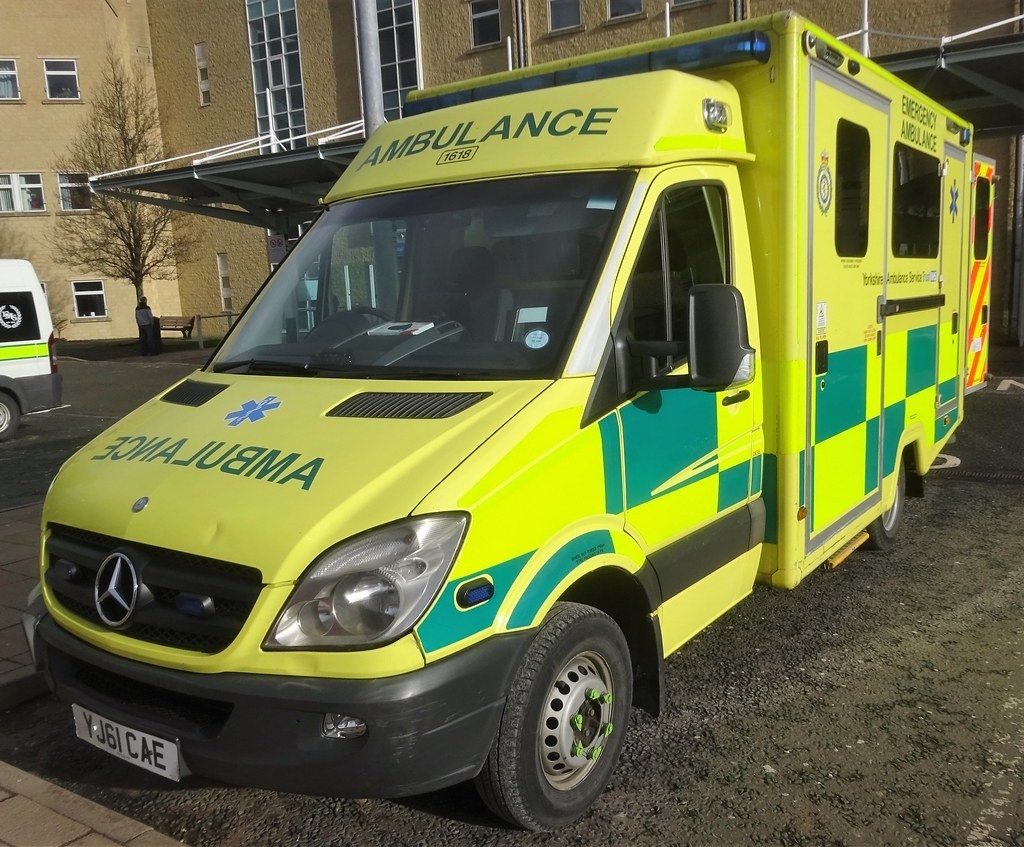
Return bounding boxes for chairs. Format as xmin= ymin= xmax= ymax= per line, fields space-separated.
xmin=640 ymin=232 xmax=687 ymax=271
xmin=413 ymin=246 xmax=494 ymax=301
xmin=512 ymin=235 xmax=600 ymax=282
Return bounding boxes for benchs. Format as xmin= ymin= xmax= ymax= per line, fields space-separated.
xmin=160 ymin=316 xmax=195 ymax=337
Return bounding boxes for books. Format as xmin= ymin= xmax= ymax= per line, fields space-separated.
xmin=367 ymin=322 xmax=434 ymax=336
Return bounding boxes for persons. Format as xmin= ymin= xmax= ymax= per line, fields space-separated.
xmin=135 ymin=297 xmax=159 ymax=356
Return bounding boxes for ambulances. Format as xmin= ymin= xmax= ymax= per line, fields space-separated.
xmin=19 ymin=8 xmax=1002 ymax=833
xmin=0 ymin=258 xmax=63 ymax=443
